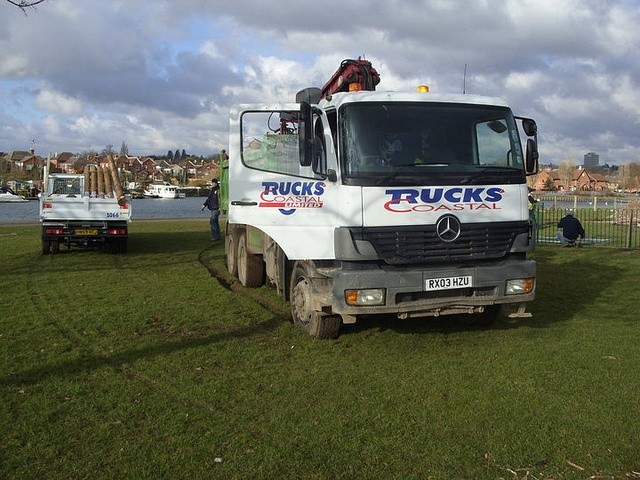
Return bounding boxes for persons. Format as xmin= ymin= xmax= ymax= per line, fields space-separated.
xmin=202 ymin=178 xmax=220 ymax=241
xmin=527 ymin=186 xmax=540 ymax=240
xmin=558 ymin=212 xmax=584 ymax=247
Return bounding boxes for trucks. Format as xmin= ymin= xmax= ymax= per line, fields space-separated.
xmin=37 ymin=165 xmax=133 ymax=254
xmin=218 ymin=57 xmax=540 ymax=340
